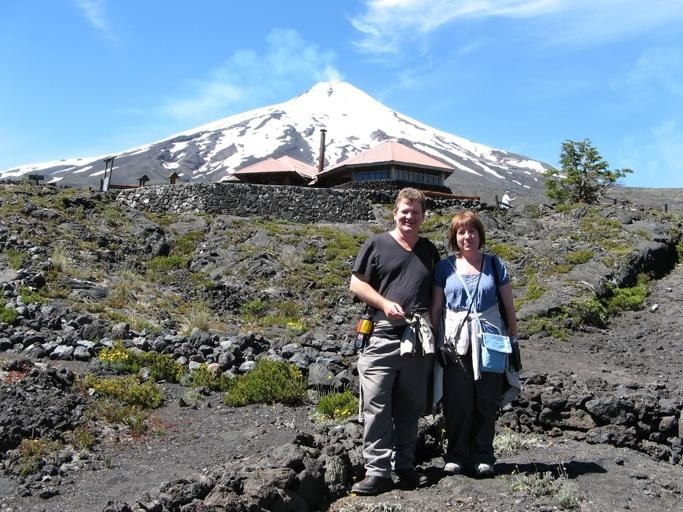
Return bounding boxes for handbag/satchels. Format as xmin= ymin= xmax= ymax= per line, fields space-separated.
xmin=356 ymin=313 xmax=374 ymax=348
xmin=511 ymin=339 xmax=523 ymax=372
xmin=479 ymin=334 xmax=511 ymax=374
xmin=438 ymin=342 xmax=466 ymax=378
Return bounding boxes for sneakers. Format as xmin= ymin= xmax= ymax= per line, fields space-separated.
xmin=398 ymin=473 xmax=428 ymax=485
xmin=351 ymin=475 xmax=392 ymax=495
xmin=474 ymin=462 xmax=494 ymax=475
xmin=443 ymin=462 xmax=463 ymax=473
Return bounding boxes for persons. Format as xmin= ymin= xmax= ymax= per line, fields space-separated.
xmin=349 ymin=186 xmax=440 ymax=495
xmin=432 ymin=211 xmax=524 ymax=479
xmin=501 ymin=190 xmax=517 ymax=209
